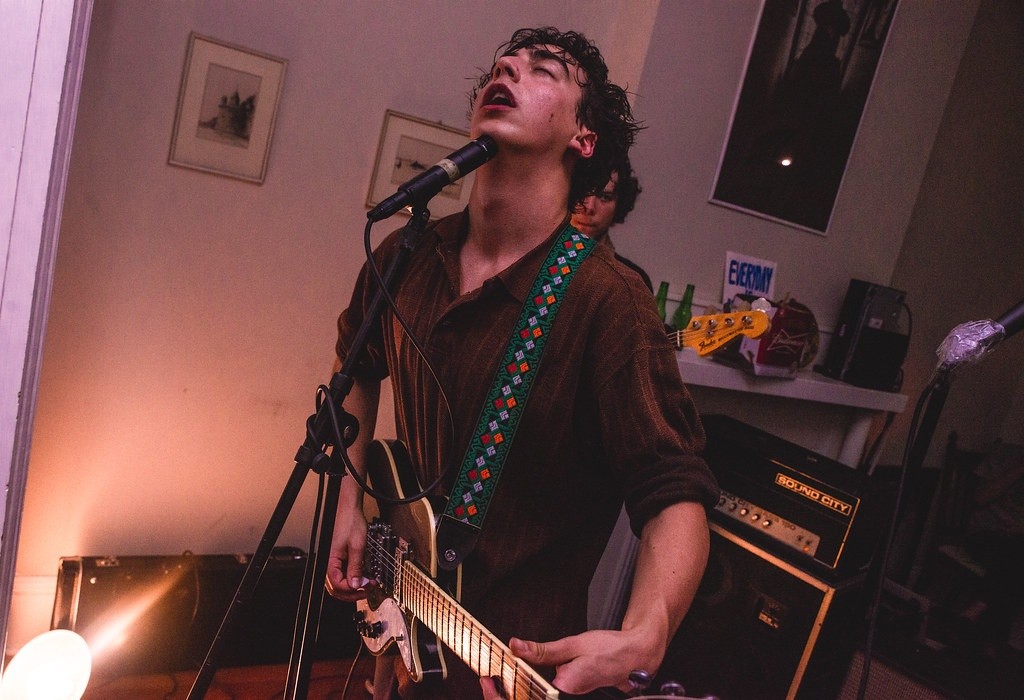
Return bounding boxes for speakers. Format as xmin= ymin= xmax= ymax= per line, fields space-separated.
xmin=662 ymin=518 xmax=873 ymax=700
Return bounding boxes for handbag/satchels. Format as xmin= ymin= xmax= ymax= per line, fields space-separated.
xmin=713 ymin=289 xmax=820 ymax=381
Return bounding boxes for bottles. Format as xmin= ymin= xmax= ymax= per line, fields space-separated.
xmin=671 ymin=284 xmax=696 ymax=350
xmin=655 ymin=281 xmax=670 ymax=322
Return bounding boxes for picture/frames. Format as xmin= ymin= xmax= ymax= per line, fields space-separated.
xmin=708 ymin=0 xmax=901 ymax=240
xmin=167 ymin=31 xmax=288 ymax=187
xmin=366 ymin=109 xmax=476 ymax=223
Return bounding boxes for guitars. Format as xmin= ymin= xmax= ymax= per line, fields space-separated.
xmin=351 ymin=436 xmax=715 ymax=700
xmin=666 ymin=294 xmax=774 ymax=359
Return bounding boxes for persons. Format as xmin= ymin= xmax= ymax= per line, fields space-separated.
xmin=323 ymin=26 xmax=719 ymax=700
xmin=569 ymin=158 xmax=653 ymax=294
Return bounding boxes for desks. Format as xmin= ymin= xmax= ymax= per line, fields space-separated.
xmin=587 ymin=350 xmax=908 ymax=632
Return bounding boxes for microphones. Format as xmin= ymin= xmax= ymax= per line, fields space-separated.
xmin=365 ymin=131 xmax=501 ymax=223
